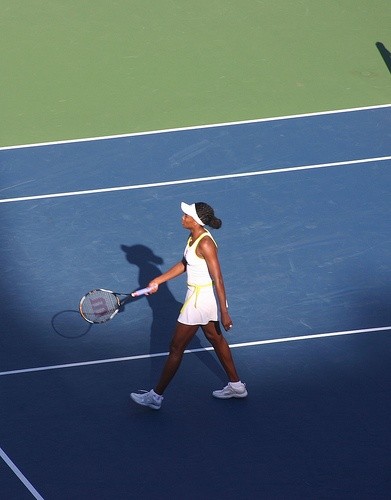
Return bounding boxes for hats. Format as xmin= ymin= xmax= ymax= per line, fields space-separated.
xmin=180 ymin=201 xmax=206 ymax=227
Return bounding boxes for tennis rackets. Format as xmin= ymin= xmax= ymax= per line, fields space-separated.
xmin=79 ymin=283 xmax=158 ymax=324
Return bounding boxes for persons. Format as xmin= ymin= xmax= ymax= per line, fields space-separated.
xmin=131 ymin=201 xmax=247 ymax=410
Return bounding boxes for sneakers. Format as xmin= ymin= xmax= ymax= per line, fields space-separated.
xmin=213 ymin=382 xmax=248 ymax=398
xmin=130 ymin=389 xmax=162 ymax=410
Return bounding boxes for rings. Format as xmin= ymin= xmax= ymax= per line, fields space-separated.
xmin=229 ymin=325 xmax=232 ymax=328
